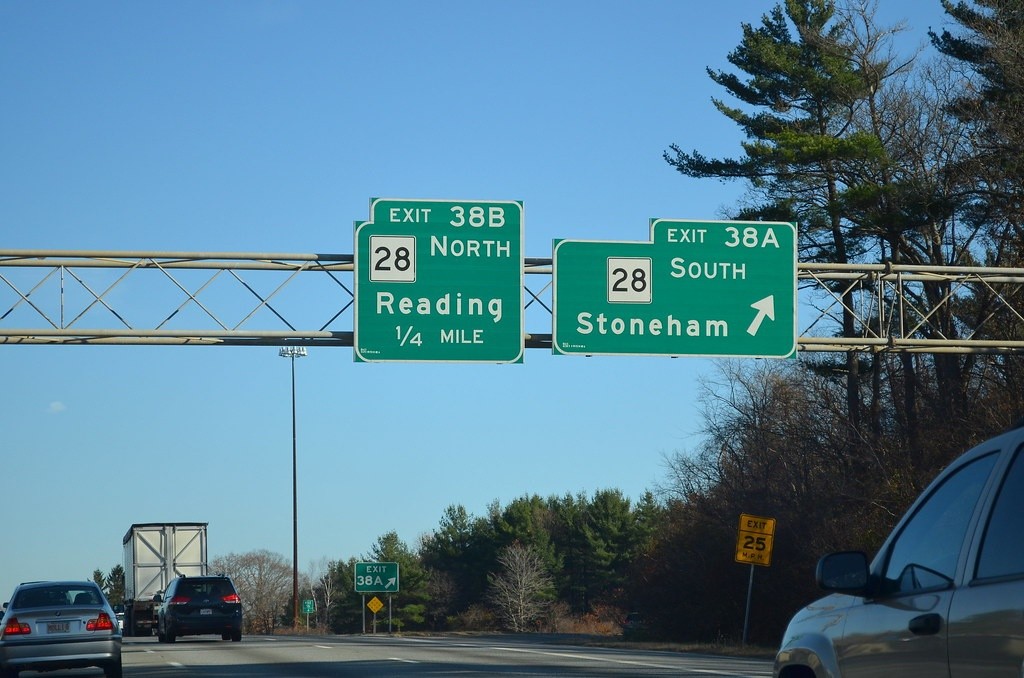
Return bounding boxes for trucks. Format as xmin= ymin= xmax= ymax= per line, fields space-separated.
xmin=122 ymin=522 xmax=208 ymax=638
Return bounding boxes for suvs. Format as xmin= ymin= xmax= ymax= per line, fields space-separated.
xmin=771 ymin=425 xmax=1024 ymax=678
xmin=153 ymin=573 xmax=243 ymax=643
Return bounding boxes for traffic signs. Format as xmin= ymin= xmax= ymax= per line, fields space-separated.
xmin=352 ymin=196 xmax=525 ymax=365
xmin=354 ymin=561 xmax=400 ymax=593
xmin=303 ymin=599 xmax=314 ymax=614
xmin=549 ymin=217 xmax=799 ymax=361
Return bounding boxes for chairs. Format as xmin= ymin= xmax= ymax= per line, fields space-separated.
xmin=73 ymin=593 xmax=92 ymax=604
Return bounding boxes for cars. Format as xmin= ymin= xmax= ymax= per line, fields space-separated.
xmin=114 ymin=612 xmax=124 ymax=630
xmin=0 ymin=577 xmax=126 ymax=678
